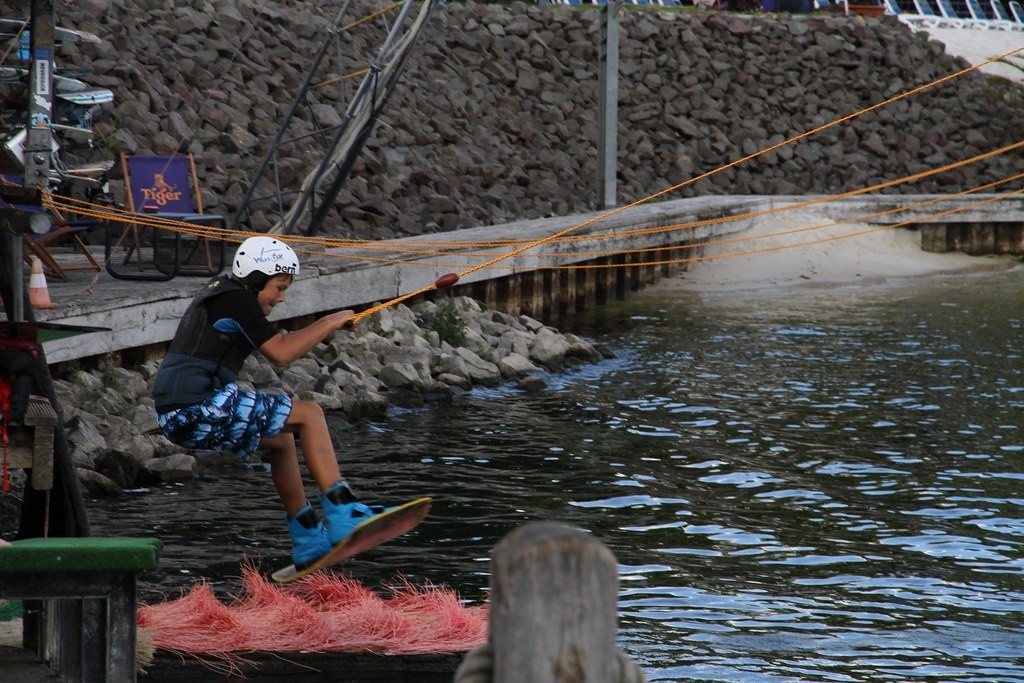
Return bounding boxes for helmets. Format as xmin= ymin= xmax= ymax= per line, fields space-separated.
xmin=232 ymin=236 xmax=300 ymax=278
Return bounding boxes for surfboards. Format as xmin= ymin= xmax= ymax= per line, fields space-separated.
xmin=270 ymin=496 xmax=433 ymax=582
xmin=0 ymin=19 xmax=114 ymax=188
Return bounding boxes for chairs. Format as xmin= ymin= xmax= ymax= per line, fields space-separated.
xmin=104 ymin=151 xmax=228 ymax=282
xmin=0 ymin=172 xmax=102 ymax=283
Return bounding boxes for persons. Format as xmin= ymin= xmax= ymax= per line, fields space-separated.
xmin=152 ymin=237 xmax=397 ymax=572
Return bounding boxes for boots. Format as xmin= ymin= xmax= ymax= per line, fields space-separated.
xmin=318 ymin=481 xmax=396 ymax=544
xmin=285 ymin=499 xmax=333 ymax=571
